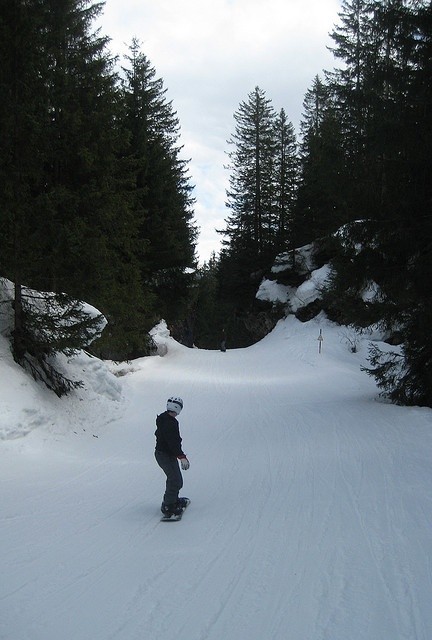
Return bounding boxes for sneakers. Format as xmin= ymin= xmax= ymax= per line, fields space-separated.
xmin=161 ymin=502 xmax=183 ymax=516
xmin=178 ymin=497 xmax=190 ymax=507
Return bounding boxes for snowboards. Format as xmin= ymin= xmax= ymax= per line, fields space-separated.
xmin=160 ymin=497 xmax=190 ymax=522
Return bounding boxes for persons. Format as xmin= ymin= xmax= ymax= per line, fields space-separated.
xmin=154 ymin=395 xmax=190 ymax=517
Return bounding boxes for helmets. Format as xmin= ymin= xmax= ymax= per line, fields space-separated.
xmin=167 ymin=396 xmax=183 ymax=415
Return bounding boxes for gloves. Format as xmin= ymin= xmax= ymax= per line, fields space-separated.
xmin=178 ymin=455 xmax=189 ymax=470
